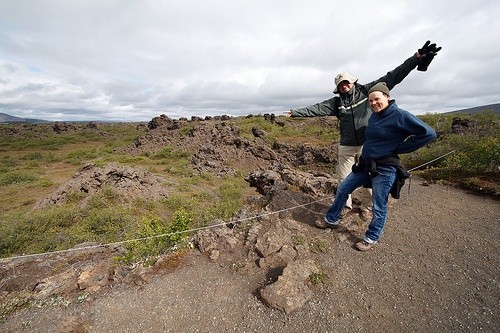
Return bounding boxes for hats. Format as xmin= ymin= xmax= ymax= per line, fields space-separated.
xmin=368 ymin=82 xmax=390 ymax=97
xmin=332 ymin=74 xmax=358 ymax=94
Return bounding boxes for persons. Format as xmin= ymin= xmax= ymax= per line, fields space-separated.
xmin=282 ymin=50 xmax=426 ymax=221
xmin=315 ymin=82 xmax=436 ymax=250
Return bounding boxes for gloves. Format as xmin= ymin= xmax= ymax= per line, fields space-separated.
xmin=417 ymin=40 xmax=442 ymax=71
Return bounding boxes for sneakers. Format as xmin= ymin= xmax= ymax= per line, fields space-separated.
xmin=355 ymin=240 xmax=377 ymax=251
xmin=315 ymin=219 xmax=338 ymax=229
xmin=359 ymin=211 xmax=372 ymax=222
xmin=338 ymin=207 xmax=351 ymax=219
